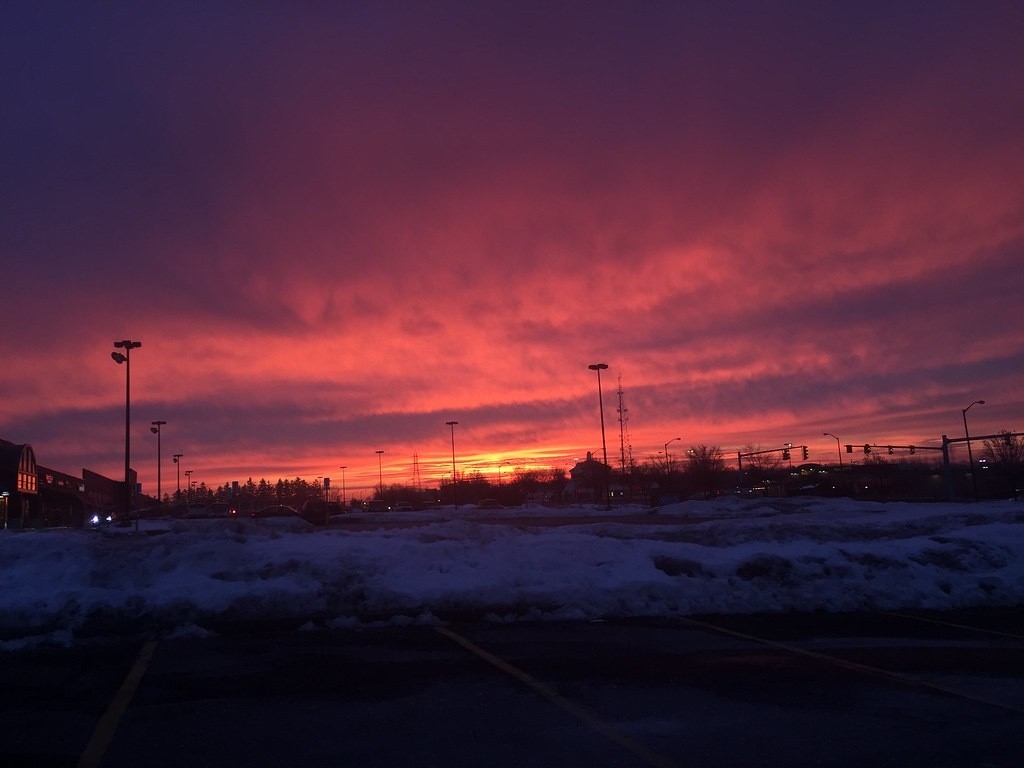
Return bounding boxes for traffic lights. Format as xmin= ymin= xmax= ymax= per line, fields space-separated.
xmin=782 ymin=449 xmax=790 ymax=460
xmin=802 ymin=445 xmax=809 ymax=461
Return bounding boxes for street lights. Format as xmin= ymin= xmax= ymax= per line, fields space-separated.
xmin=498 ymin=463 xmax=510 ymax=497
xmin=375 ymin=451 xmax=384 ymax=493
xmin=591 ymin=447 xmax=604 ymax=461
xmin=659 ymin=437 xmax=682 ymax=494
xmin=110 ymin=340 xmax=141 ymax=525
xmin=963 ymin=399 xmax=986 ymax=501
xmin=824 ymin=433 xmax=843 ymax=475
xmin=341 ymin=466 xmax=346 ymax=504
xmin=151 ymin=422 xmax=167 ymax=509
xmin=186 ymin=470 xmax=192 ymax=491
xmin=191 ymin=481 xmax=197 ymax=491
xmin=172 ymin=453 xmax=182 ymax=499
xmin=446 ymin=422 xmax=459 ymax=509
xmin=588 ymin=364 xmax=612 ymax=510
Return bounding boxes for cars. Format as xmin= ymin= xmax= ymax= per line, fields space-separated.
xmin=391 ymin=501 xmax=414 ymax=512
xmin=327 ymin=502 xmax=345 ymax=514
xmin=188 ymin=502 xmax=207 ymax=517
xmin=207 ymin=502 xmax=230 ymax=518
xmin=478 ymin=499 xmax=503 ymax=510
xmin=251 ymin=505 xmax=298 ymax=518
xmin=368 ymin=500 xmax=390 ymax=513
xmin=302 ymin=497 xmax=329 ymax=519
